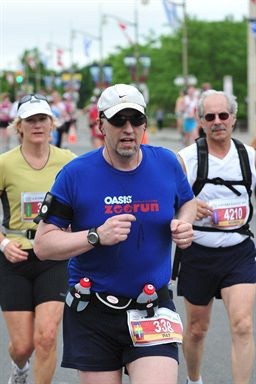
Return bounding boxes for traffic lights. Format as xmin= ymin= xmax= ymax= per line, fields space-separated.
xmin=16 ymin=70 xmax=25 ymax=85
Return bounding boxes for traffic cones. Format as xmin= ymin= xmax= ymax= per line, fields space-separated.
xmin=67 ymin=124 xmax=77 ymax=144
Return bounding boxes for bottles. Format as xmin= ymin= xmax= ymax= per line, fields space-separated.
xmin=65 ymin=277 xmax=91 ymax=312
xmin=135 ymin=284 xmax=158 ymax=317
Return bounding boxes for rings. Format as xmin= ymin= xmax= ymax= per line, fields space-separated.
xmin=184 ymin=239 xmax=186 ymax=243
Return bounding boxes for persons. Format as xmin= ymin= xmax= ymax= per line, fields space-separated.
xmin=176 ymin=91 xmax=256 ymax=384
xmin=0 ymin=94 xmax=77 ymax=384
xmin=0 ymin=82 xmax=211 ymax=149
xmin=32 ymin=81 xmax=197 ymax=384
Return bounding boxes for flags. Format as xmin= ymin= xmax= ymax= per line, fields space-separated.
xmin=44 ymin=56 xmax=152 ymax=103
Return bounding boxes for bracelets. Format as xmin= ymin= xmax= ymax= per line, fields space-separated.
xmin=0 ymin=238 xmax=11 ymax=252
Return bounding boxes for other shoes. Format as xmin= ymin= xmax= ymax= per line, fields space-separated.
xmin=7 ymin=361 xmax=30 ymax=384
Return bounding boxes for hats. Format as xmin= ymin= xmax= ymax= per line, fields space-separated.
xmin=97 ymin=84 xmax=147 ymax=119
xmin=17 ymin=96 xmax=54 ymax=119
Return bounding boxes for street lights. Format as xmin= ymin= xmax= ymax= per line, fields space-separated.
xmin=140 ymin=0 xmax=188 ymax=92
xmin=100 ymin=5 xmax=140 ymax=88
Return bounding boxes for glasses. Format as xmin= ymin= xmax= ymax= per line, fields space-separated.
xmin=100 ymin=115 xmax=147 ymax=127
xmin=201 ymin=111 xmax=232 ymax=122
xmin=17 ymin=93 xmax=49 ymax=109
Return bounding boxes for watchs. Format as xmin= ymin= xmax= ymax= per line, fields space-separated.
xmin=86 ymin=228 xmax=102 ymax=248
